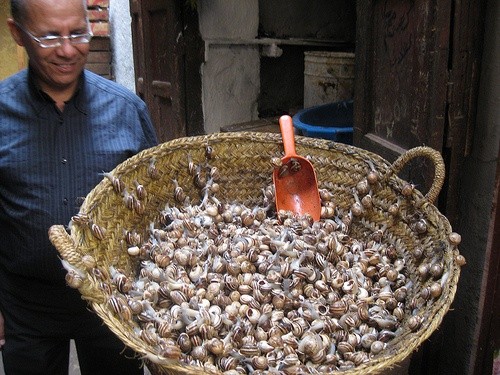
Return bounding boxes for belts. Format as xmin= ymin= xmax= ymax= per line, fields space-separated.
xmin=13 ymin=274 xmax=66 ymax=287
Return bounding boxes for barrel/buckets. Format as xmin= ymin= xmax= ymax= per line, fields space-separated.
xmin=292 ymin=100 xmax=353 ymax=146
xmin=303 ymin=51 xmax=354 ymax=111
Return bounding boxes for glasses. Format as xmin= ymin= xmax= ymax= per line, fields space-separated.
xmin=15 ymin=16 xmax=93 ymax=48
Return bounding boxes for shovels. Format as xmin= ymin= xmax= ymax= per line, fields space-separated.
xmin=273 ymin=115 xmax=321 ymax=223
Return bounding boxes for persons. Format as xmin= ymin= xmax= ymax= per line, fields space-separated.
xmin=0 ymin=1 xmax=160 ymax=374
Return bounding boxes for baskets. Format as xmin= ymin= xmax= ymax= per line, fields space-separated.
xmin=47 ymin=130 xmax=461 ymax=375
